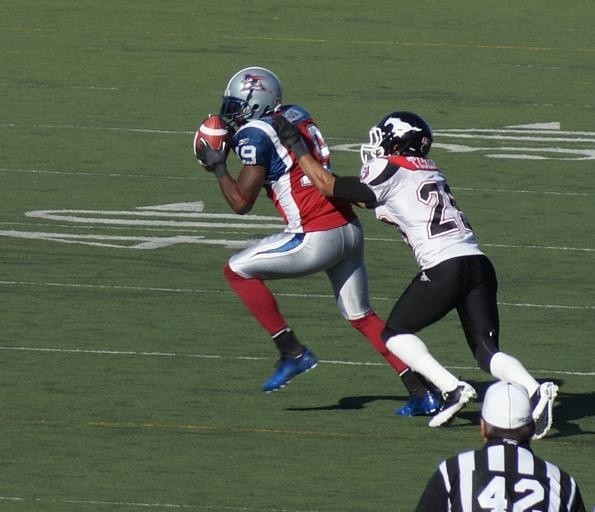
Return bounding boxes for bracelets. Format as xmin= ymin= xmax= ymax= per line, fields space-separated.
xmin=290 ymin=139 xmax=310 ymax=161
xmin=214 ymin=165 xmax=230 ymax=179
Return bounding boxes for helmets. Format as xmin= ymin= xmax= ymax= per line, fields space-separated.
xmin=220 ymin=67 xmax=282 ymax=123
xmin=375 ymin=111 xmax=431 ymax=157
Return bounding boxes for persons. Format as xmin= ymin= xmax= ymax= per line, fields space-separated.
xmin=413 ymin=378 xmax=588 ymax=512
xmin=272 ymin=107 xmax=560 ymax=440
xmin=191 ymin=64 xmax=444 ymax=418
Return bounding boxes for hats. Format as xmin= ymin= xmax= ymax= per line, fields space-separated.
xmin=482 ymin=380 xmax=534 ymax=430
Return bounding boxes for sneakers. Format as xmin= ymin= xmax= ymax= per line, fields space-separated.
xmin=428 ymin=379 xmax=478 ymax=428
xmin=260 ymin=346 xmax=317 ymax=393
xmin=529 ymin=380 xmax=559 ymax=442
xmin=396 ymin=392 xmax=436 ymax=417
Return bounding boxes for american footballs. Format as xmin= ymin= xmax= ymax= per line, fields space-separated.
xmin=193 ymin=115 xmax=235 ymax=173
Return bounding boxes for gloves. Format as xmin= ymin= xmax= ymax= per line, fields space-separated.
xmin=196 ymin=138 xmax=227 ymax=176
xmin=270 ymin=114 xmax=309 ymax=160
